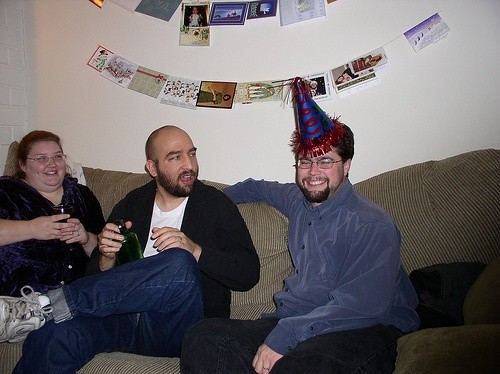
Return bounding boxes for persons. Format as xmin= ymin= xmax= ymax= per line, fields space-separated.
xmin=188 ymin=7 xmax=203 ymax=27
xmin=0 ymin=124 xmax=261 ymax=374
xmin=0 ymin=131 xmax=106 ymax=298
xmin=197 ymin=85 xmax=231 ymax=104
xmin=309 ymin=80 xmax=322 ymax=97
xmin=180 ymin=77 xmax=421 ymax=374
xmin=335 ymin=54 xmax=383 ymax=84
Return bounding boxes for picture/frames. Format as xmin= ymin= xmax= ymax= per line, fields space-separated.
xmin=208 ymin=2 xmax=250 ymax=25
xmin=305 ymin=70 xmax=332 ymax=101
xmin=182 ymin=2 xmax=211 ymax=29
xmin=196 ymin=80 xmax=237 ymax=110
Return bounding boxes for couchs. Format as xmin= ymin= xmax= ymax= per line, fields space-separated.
xmin=0 ymin=140 xmax=500 ymax=374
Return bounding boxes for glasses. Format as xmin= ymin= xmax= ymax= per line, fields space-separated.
xmin=298 ymin=157 xmax=345 ymax=169
xmin=26 ymin=154 xmax=66 ymax=162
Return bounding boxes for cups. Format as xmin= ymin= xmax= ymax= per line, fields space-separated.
xmin=52 ymin=205 xmax=74 ymax=224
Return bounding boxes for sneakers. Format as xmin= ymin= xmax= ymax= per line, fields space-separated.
xmin=0 ymin=285 xmax=53 ymax=343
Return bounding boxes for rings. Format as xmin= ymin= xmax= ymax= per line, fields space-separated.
xmin=178 ymin=236 xmax=182 ymax=242
xmin=77 ymin=231 xmax=79 ymax=236
xmin=263 ymin=367 xmax=269 ymax=370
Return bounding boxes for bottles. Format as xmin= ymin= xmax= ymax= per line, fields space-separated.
xmin=114 ymin=219 xmax=143 ymax=265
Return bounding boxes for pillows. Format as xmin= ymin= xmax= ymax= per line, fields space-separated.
xmin=408 ymin=262 xmax=488 ymax=329
